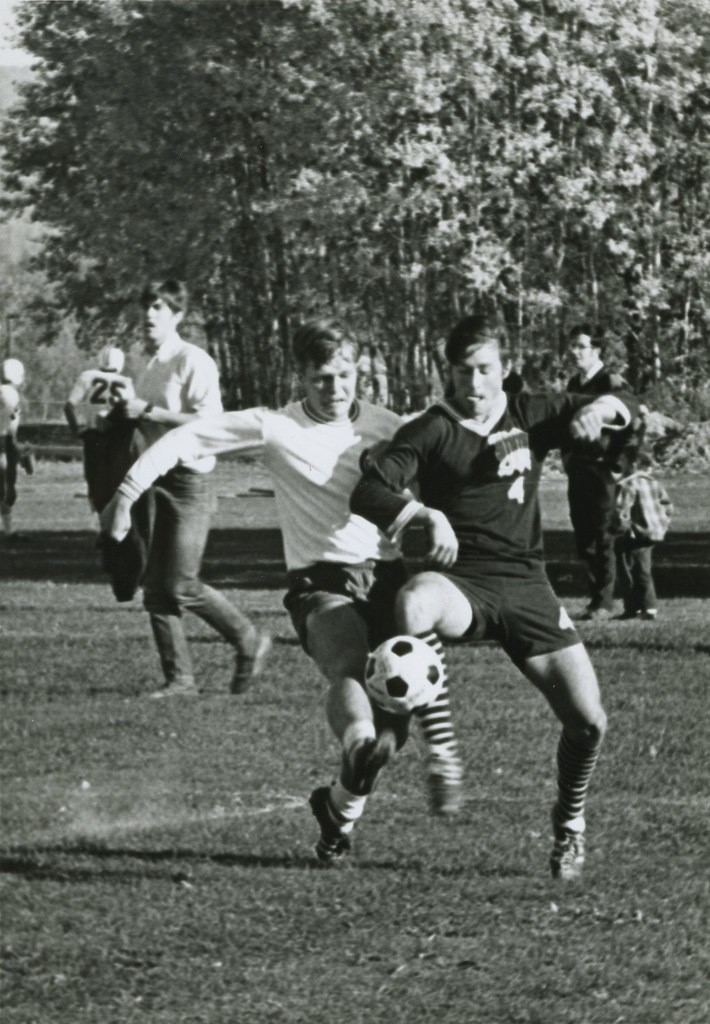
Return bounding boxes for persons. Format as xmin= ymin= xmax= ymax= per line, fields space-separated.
xmin=0 ymin=357 xmax=33 ymax=537
xmin=65 ymin=344 xmax=142 ymax=548
xmin=95 ymin=309 xmax=439 ymax=873
xmin=97 ymin=277 xmax=274 ymax=703
xmin=544 ymin=321 xmax=672 ymax=624
xmin=348 ymin=311 xmax=643 ymax=883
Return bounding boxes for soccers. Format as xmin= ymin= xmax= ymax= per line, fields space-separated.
xmin=365 ymin=634 xmax=446 ymax=714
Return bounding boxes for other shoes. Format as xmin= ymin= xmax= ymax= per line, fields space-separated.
xmin=426 ymin=760 xmax=467 ymax=816
xmin=549 ymin=808 xmax=587 ymax=884
xmin=619 ymin=608 xmax=656 ymax=621
xmin=148 ymin=678 xmax=199 ymax=698
xmin=232 ymin=624 xmax=276 ymax=695
xmin=578 ymin=607 xmax=613 ymax=620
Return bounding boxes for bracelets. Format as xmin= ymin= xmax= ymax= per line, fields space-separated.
xmin=137 ymin=400 xmax=156 ymax=422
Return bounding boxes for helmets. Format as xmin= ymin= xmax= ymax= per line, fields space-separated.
xmin=98 ymin=346 xmax=125 ymax=374
xmin=1 ymin=359 xmax=24 ymax=385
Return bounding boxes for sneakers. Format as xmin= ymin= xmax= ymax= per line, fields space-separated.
xmin=309 ymin=784 xmax=353 ymax=866
xmin=344 ymin=730 xmax=397 ymax=793
